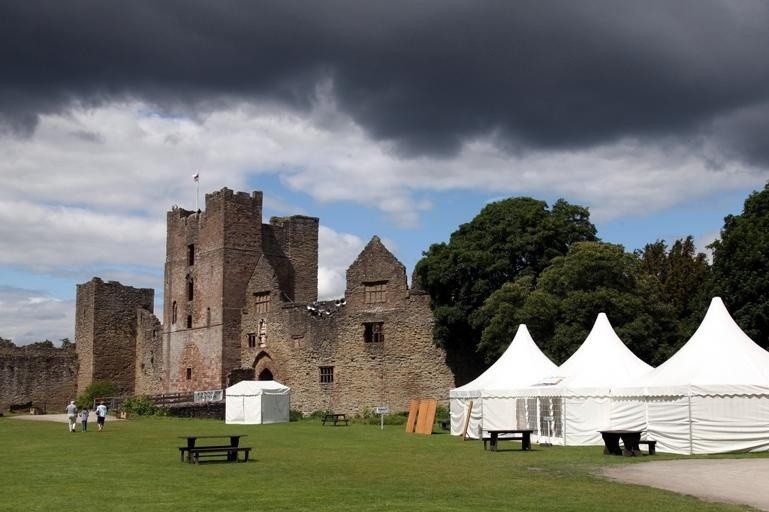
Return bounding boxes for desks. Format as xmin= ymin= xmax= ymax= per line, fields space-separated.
xmin=176 ymin=435 xmax=248 ymax=457
xmin=488 ymin=430 xmax=538 ymax=451
xmin=325 ymin=414 xmax=345 ymax=420
xmin=597 ymin=430 xmax=643 ymax=456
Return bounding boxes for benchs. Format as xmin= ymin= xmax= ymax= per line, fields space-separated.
xmin=619 ymin=441 xmax=656 ymax=456
xmin=190 ymin=448 xmax=251 ymax=464
xmin=482 ymin=437 xmax=522 ymax=450
xmin=176 ymin=446 xmax=230 ymax=462
xmin=334 ymin=420 xmax=349 ymax=426
xmin=322 ymin=420 xmax=338 ymax=426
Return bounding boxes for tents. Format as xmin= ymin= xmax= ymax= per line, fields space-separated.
xmin=530 ymin=310 xmax=655 ymax=447
xmin=223 ymin=379 xmax=291 ymax=425
xmin=646 ymin=295 xmax=768 ymax=457
xmin=449 ymin=324 xmax=559 ymax=439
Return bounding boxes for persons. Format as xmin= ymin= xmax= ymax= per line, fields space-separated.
xmin=64 ymin=400 xmax=78 ymax=432
xmin=94 ymin=400 xmax=108 ymax=432
xmin=78 ymin=406 xmax=89 ymax=432
